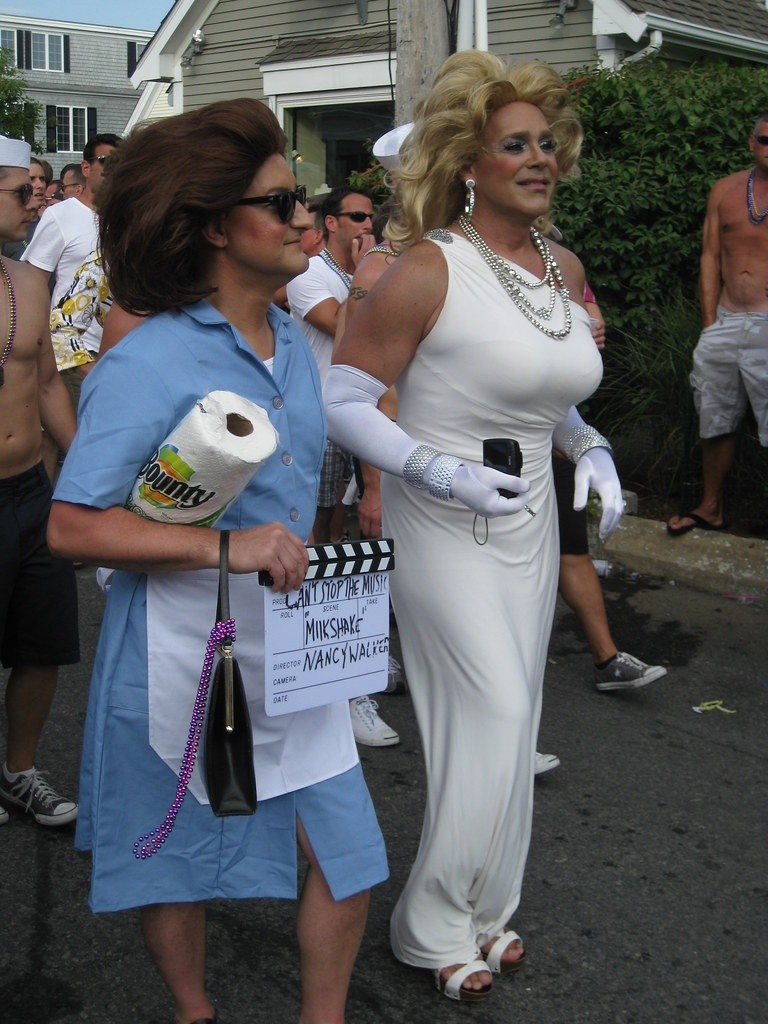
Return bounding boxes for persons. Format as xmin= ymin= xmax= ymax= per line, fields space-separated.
xmin=666 ymin=118 xmax=768 ymax=535
xmin=275 ymin=185 xmax=667 ymax=775
xmin=24 ymin=132 xmax=154 ymax=567
xmin=319 ymin=49 xmax=623 ymax=1004
xmin=49 ymin=97 xmax=390 ymax=1023
xmin=0 ymin=136 xmax=81 ymax=827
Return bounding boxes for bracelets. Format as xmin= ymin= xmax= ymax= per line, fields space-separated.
xmin=564 ymin=425 xmax=613 ymax=462
xmin=403 ymin=445 xmax=440 ymax=490
xmin=429 ymin=454 xmax=464 ymax=501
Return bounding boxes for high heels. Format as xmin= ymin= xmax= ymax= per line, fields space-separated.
xmin=430 ymin=944 xmax=495 ymax=1004
xmin=473 ymin=925 xmax=528 ymax=974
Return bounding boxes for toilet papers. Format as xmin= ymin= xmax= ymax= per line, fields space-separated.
xmin=124 ymin=389 xmax=280 ymax=571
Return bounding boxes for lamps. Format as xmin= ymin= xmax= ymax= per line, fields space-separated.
xmin=292 ymin=149 xmax=305 ymax=163
xmin=549 ymin=0 xmax=576 ymax=30
xmin=180 ymin=30 xmax=205 ymax=68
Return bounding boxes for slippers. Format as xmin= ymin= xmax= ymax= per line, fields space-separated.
xmin=666 ymin=509 xmax=726 ymax=537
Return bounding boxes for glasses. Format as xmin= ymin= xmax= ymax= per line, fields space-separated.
xmin=233 ymin=183 xmax=308 ymax=222
xmin=59 ymin=182 xmax=78 ymax=192
xmin=0 ymin=183 xmax=34 ymax=206
xmin=86 ymin=153 xmax=107 ymax=165
xmin=752 ymin=133 xmax=768 ymax=146
xmin=335 ymin=210 xmax=373 ymax=222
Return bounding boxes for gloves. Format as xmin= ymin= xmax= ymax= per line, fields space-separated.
xmin=550 ymin=402 xmax=625 ymax=543
xmin=320 ymin=362 xmax=531 ymax=518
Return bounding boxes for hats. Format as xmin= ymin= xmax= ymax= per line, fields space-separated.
xmin=0 ymin=134 xmax=33 ymax=172
xmin=370 ymin=118 xmax=417 ymax=171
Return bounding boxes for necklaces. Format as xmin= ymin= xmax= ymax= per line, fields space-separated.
xmin=746 ymin=167 xmax=768 ymax=225
xmin=460 ymin=216 xmax=572 ymax=341
xmin=0 ymin=256 xmax=17 ymax=387
xmin=318 ymin=247 xmax=352 ymax=291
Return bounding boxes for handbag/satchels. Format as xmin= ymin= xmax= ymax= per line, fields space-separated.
xmin=134 ymin=528 xmax=259 ymax=859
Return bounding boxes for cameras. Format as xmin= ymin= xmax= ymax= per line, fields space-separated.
xmin=482 ymin=438 xmax=524 ymax=499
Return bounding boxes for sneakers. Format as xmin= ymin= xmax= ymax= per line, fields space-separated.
xmin=529 ymin=751 xmax=560 ymax=779
xmin=593 ymin=650 xmax=670 ymax=692
xmin=0 ymin=804 xmax=11 ymax=827
xmin=346 ymin=695 xmax=402 ymax=749
xmin=377 ymin=655 xmax=409 ymax=696
xmin=0 ymin=762 xmax=78 ymax=827
